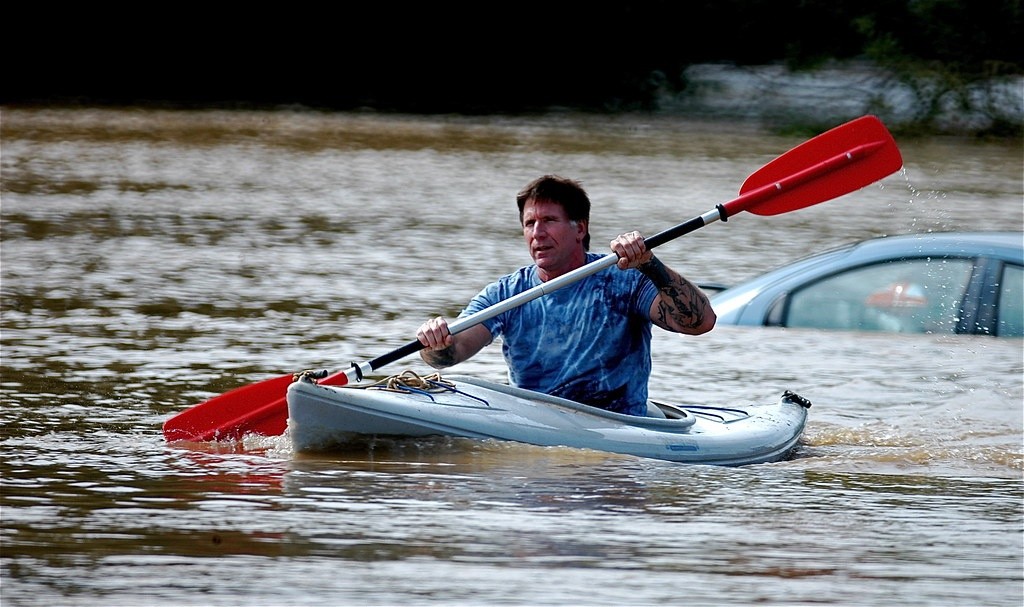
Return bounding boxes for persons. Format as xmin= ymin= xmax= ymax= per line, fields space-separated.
xmin=415 ymin=172 xmax=718 ymax=419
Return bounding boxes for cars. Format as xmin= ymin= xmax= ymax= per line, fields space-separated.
xmin=706 ymin=234 xmax=1024 ymax=335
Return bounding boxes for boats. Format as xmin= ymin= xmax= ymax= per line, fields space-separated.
xmin=285 ymin=375 xmax=811 ymax=468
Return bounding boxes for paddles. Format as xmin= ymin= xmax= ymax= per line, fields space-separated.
xmin=159 ymin=113 xmax=908 ymax=455
